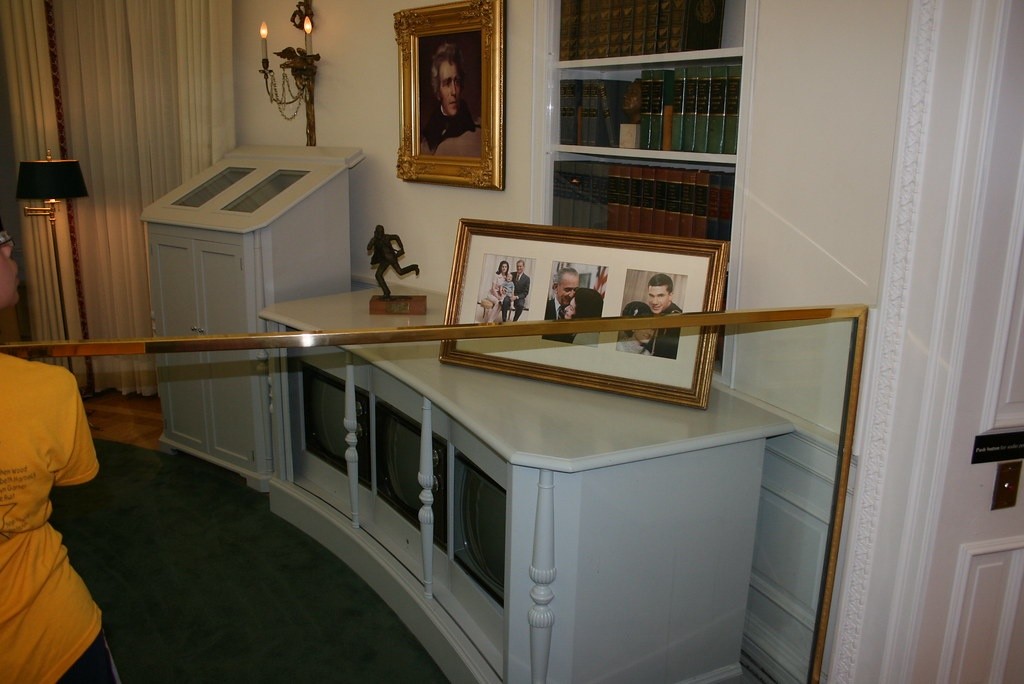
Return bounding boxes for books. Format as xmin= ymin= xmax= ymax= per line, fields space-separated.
xmin=552 ymin=159 xmax=735 ymax=242
xmin=560 ymin=65 xmax=743 ymax=155
xmin=559 ymin=0 xmax=726 ymax=61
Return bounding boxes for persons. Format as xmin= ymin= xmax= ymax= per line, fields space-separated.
xmin=642 ymin=273 xmax=683 ymax=360
xmin=542 ymin=267 xmax=580 ymax=349
xmin=0 ymin=218 xmax=122 ymax=684
xmin=485 ymin=259 xmax=530 ymax=323
xmin=565 ymin=288 xmax=603 ymax=349
xmin=616 ymin=300 xmax=657 ymax=357
xmin=366 ymin=224 xmax=420 ymax=302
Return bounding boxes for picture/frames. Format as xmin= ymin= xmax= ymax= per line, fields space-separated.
xmin=437 ymin=215 xmax=731 ymax=410
xmin=392 ymin=1 xmax=506 ymax=192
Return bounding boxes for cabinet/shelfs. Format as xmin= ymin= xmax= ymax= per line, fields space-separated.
xmin=256 ymin=282 xmax=797 ymax=684
xmin=137 ymin=142 xmax=368 ymax=494
xmin=532 ymin=0 xmax=759 ymax=394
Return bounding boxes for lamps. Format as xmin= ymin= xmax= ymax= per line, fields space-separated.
xmin=14 ymin=149 xmax=96 ymax=419
xmin=258 ymin=1 xmax=321 ymax=145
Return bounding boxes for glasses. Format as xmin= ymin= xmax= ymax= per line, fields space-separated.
xmin=0 ymin=230 xmax=15 ymax=247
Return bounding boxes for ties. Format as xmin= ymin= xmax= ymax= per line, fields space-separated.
xmin=517 ymin=273 xmax=520 ymax=281
xmin=558 ymin=305 xmax=565 ymax=320
